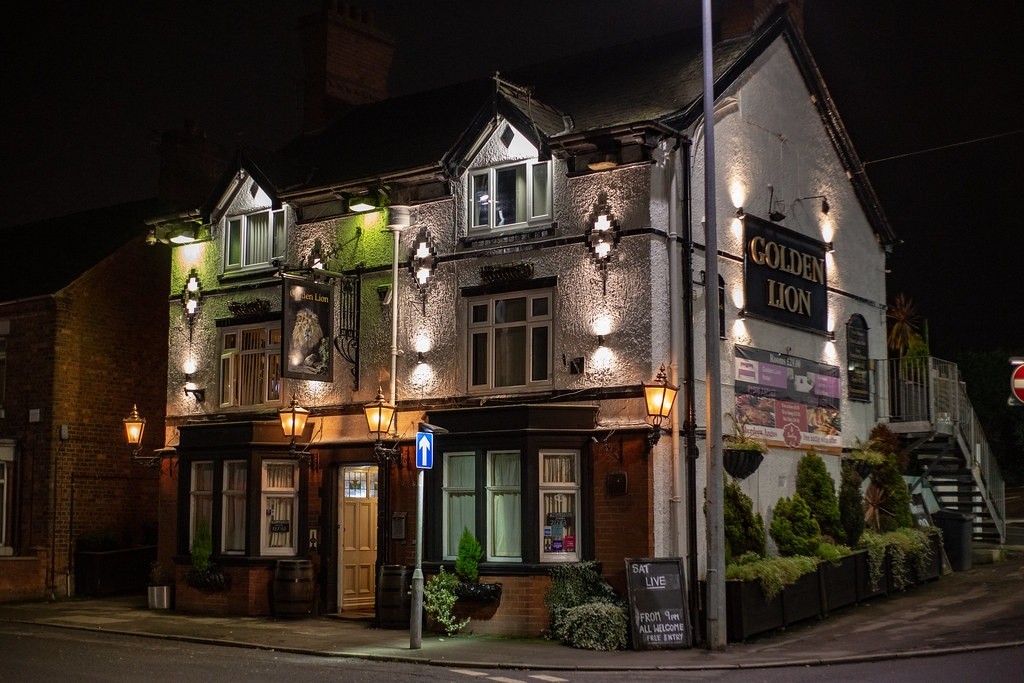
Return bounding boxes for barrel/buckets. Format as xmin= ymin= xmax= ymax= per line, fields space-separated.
xmin=274 ymin=561 xmax=316 ymax=621
xmin=376 ymin=565 xmax=415 ymax=630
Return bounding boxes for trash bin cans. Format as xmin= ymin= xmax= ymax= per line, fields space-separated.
xmin=931 ymin=510 xmax=973 ymax=572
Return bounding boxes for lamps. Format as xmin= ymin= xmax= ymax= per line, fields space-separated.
xmin=362 ymin=385 xmax=401 ymax=469
xmin=122 ymin=404 xmax=160 ymax=467
xmin=587 ymin=153 xmax=617 ymax=171
xmin=842 ymin=434 xmax=884 ymax=481
xmin=797 ymin=195 xmax=830 ymax=213
xmin=276 ymin=393 xmax=318 ymax=469
xmin=640 ymin=364 xmax=682 ymax=446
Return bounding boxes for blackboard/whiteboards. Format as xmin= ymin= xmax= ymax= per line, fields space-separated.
xmin=624 ymin=556 xmax=692 ymax=651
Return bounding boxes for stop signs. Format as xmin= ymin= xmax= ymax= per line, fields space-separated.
xmin=1012 ymin=363 xmax=1024 ymax=402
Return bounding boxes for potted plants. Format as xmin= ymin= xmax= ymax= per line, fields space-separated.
xmin=721 ymin=411 xmax=769 ymax=478
xmin=902 ymin=339 xmax=930 ymax=384
xmin=449 ymin=525 xmax=502 ymax=622
xmin=406 ymin=564 xmax=470 ymax=636
xmin=147 ymin=561 xmax=172 ymax=608
